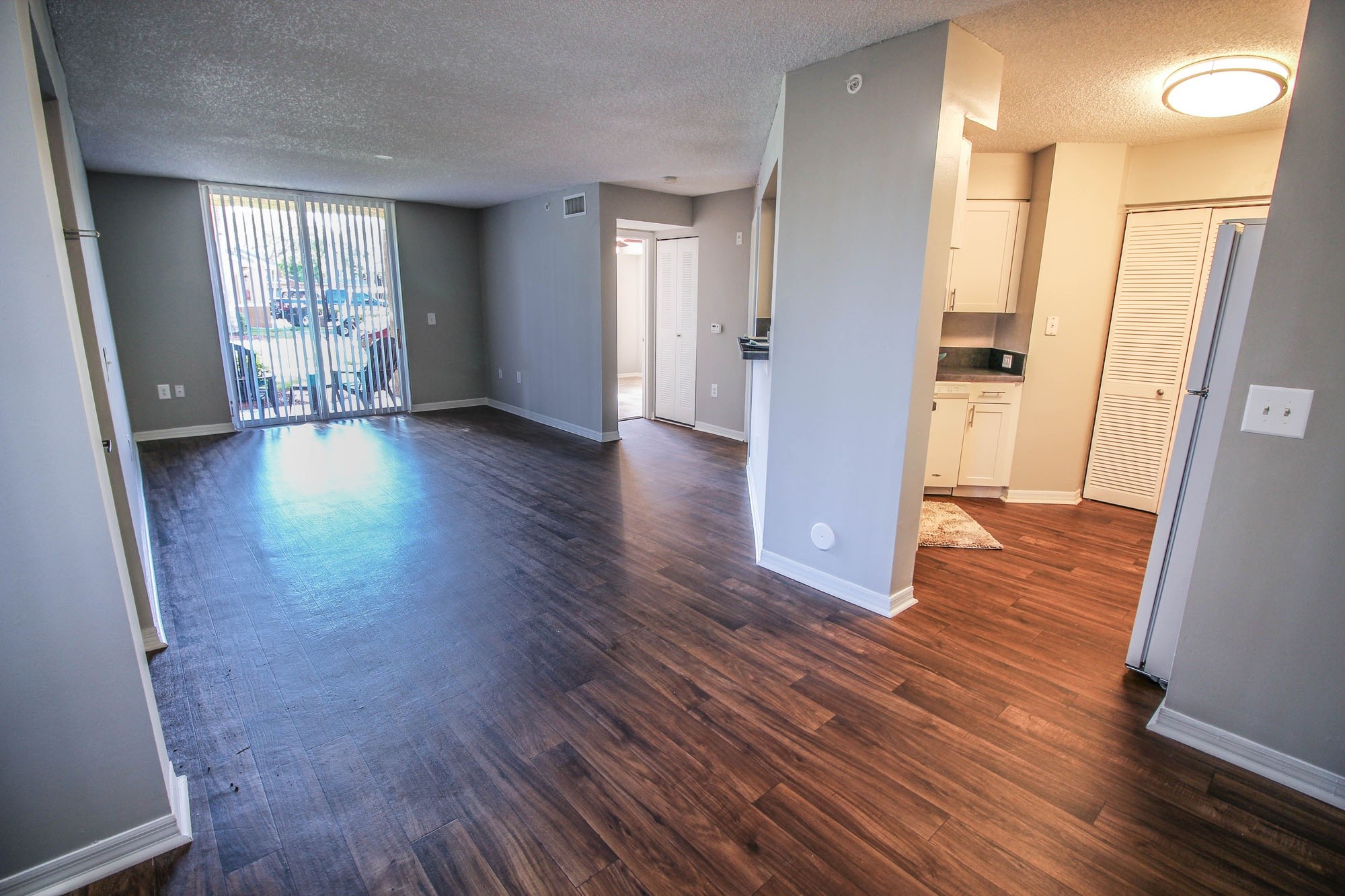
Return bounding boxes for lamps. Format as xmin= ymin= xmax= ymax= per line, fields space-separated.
xmin=1162 ymin=55 xmax=1291 ymax=117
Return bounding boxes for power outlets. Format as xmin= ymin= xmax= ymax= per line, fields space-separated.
xmin=157 ymin=385 xmax=170 ymax=399
xmin=499 ymin=368 xmax=502 ymax=379
xmin=174 ymin=385 xmax=184 ymax=397
xmin=711 ymin=383 xmax=718 ymax=398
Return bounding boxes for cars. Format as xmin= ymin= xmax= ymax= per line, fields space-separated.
xmin=269 ymin=289 xmax=392 ymax=337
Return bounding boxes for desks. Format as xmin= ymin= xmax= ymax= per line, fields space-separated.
xmin=289 ymin=379 xmax=332 ymax=415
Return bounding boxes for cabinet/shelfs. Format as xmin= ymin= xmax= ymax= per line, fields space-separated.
xmin=943 ymin=199 xmax=1031 ymax=314
xmin=957 ymin=382 xmax=1022 ymax=487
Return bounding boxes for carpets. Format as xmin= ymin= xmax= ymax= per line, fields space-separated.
xmin=918 ymin=500 xmax=1004 ymax=550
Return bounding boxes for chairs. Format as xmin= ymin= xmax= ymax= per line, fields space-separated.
xmin=230 ymin=343 xmax=277 ymax=415
xmin=333 ymin=346 xmax=397 ymax=408
